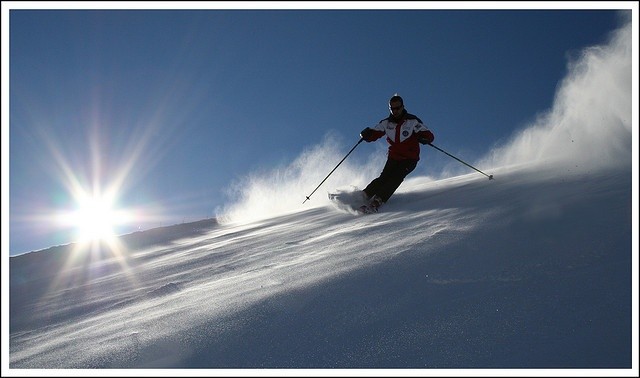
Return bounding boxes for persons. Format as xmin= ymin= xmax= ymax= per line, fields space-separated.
xmin=360 ymin=96 xmax=434 ymax=213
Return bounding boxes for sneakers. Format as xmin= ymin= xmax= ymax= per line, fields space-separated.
xmin=371 ymin=197 xmax=383 ymax=207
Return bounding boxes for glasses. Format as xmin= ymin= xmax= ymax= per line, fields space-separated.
xmin=389 ymin=104 xmax=403 ymax=110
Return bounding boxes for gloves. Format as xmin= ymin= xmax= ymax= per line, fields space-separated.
xmin=359 ymin=127 xmax=373 ymax=140
xmin=417 ymin=135 xmax=430 ymax=144
